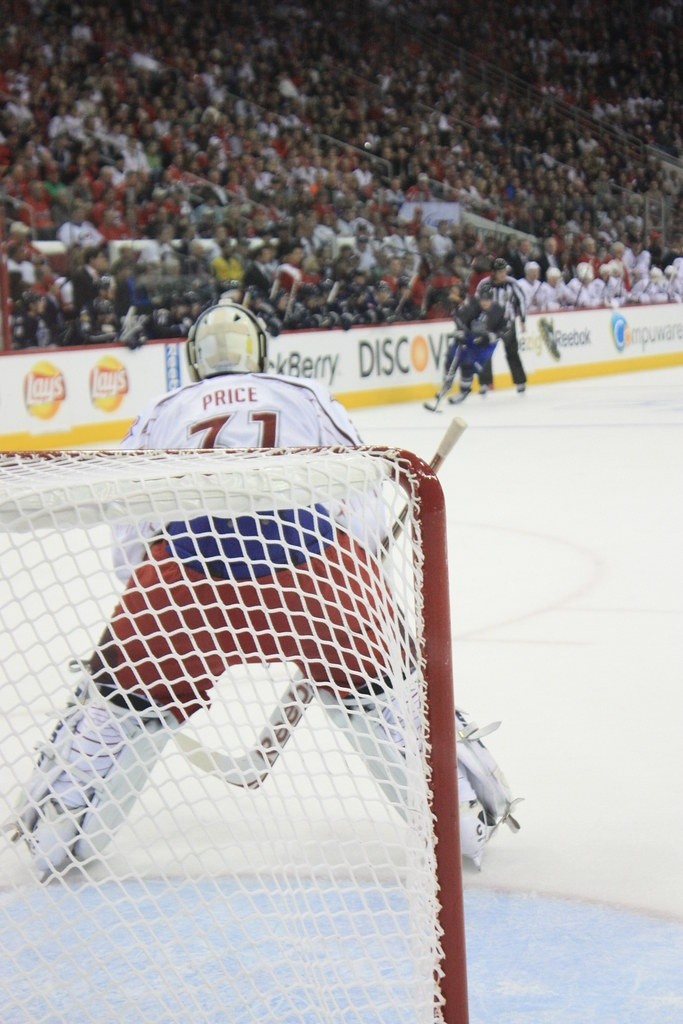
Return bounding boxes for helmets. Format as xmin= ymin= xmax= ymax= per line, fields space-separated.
xmin=524 ymin=261 xmax=677 ymax=278
xmin=492 ymin=257 xmax=511 ymax=272
xmin=185 ymin=303 xmax=269 ymax=381
xmin=479 ymin=284 xmax=493 ymax=300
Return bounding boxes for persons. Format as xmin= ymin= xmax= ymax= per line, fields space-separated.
xmin=0 ymin=0 xmax=683 ymax=350
xmin=477 ymin=258 xmax=527 ymax=396
xmin=423 ymin=283 xmax=508 ymax=413
xmin=18 ymin=302 xmax=519 ymax=882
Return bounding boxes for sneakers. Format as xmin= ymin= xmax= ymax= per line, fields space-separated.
xmin=447 ymin=382 xmax=472 ymax=395
xmin=443 ymin=376 xmax=453 ymax=389
xmin=461 ymin=799 xmax=491 ymax=872
xmin=23 ymin=799 xmax=84 ymax=884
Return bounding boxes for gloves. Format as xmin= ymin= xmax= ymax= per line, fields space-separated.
xmin=473 ymin=334 xmax=489 ymax=348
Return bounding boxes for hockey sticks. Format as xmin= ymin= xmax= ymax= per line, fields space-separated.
xmin=167 ymin=416 xmax=473 ymax=788
xmin=423 ymin=337 xmax=463 ymax=411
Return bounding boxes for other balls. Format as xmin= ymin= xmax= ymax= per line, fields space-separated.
xmin=436 ymin=410 xmax=443 ymax=414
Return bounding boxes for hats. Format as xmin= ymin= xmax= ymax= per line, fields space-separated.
xmin=11 ymin=222 xmax=30 ymax=233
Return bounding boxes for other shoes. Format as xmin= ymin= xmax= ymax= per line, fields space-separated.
xmin=478 ymin=383 xmax=493 ymax=395
xmin=515 ymin=383 xmax=526 ymax=393
